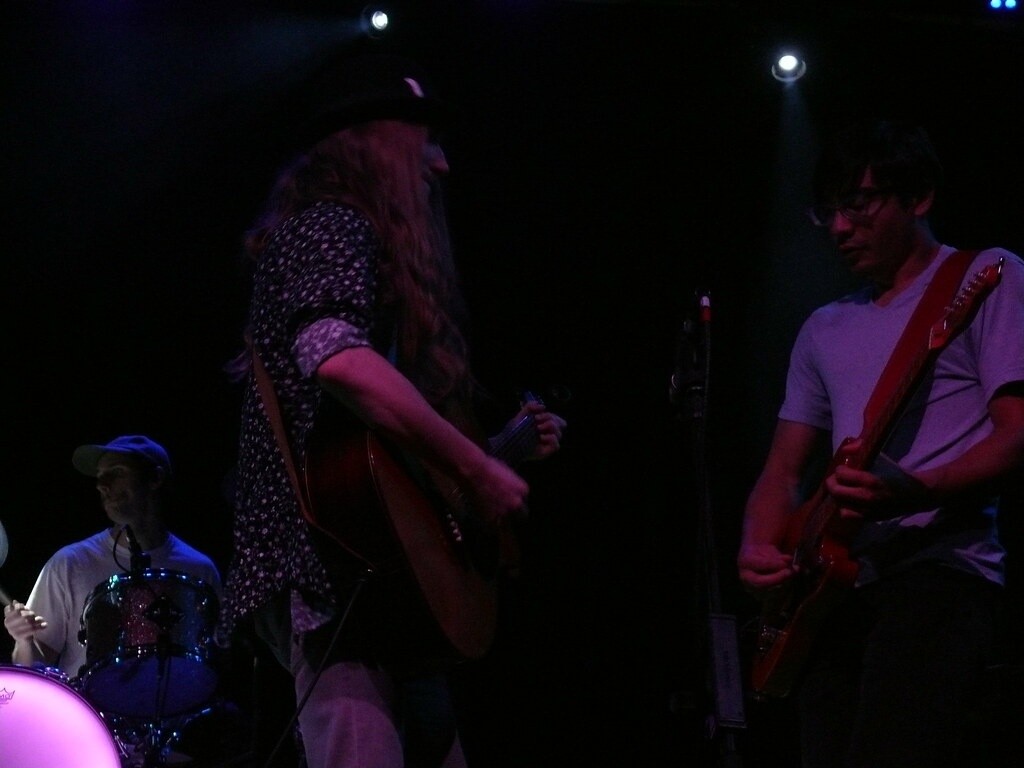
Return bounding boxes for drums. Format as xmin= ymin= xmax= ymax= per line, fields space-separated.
xmin=81 ymin=564 xmax=220 ymax=717
xmin=0 ymin=663 xmax=154 ymax=768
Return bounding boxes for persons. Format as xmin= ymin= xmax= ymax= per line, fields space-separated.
xmin=224 ymin=59 xmax=570 ymax=768
xmin=739 ymin=121 xmax=1024 ymax=768
xmin=3 ymin=434 xmax=225 ymax=768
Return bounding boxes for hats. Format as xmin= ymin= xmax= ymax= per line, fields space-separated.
xmin=71 ymin=436 xmax=172 ymax=478
xmin=297 ymin=55 xmax=446 ymax=152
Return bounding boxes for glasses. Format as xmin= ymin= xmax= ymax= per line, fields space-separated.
xmin=807 ymin=188 xmax=897 ymax=226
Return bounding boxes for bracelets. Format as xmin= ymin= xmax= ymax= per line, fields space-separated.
xmin=469 ymin=454 xmax=491 ymax=483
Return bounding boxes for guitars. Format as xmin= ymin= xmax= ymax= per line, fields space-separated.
xmin=739 ymin=255 xmax=1008 ymax=705
xmin=304 ymin=388 xmax=562 ymax=684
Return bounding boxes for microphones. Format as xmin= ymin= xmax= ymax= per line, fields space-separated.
xmin=691 ymin=282 xmax=716 ymax=326
xmin=124 ymin=524 xmax=151 ymax=572
